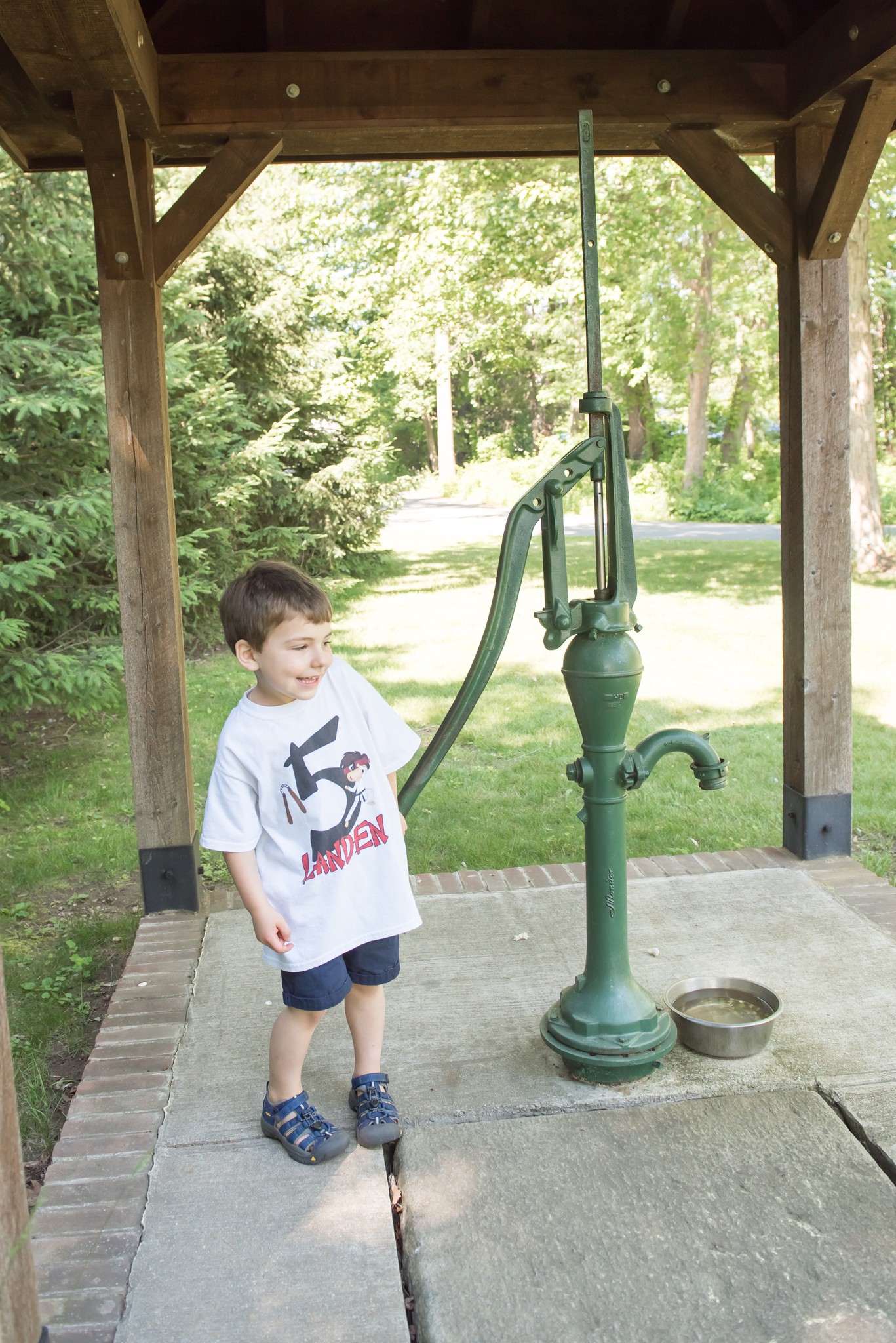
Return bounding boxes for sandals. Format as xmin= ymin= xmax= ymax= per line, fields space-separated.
xmin=348 ymin=1073 xmax=405 ymax=1147
xmin=261 ymin=1080 xmax=350 ymax=1164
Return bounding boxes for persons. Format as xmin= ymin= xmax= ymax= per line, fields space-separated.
xmin=199 ymin=561 xmax=424 ymax=1165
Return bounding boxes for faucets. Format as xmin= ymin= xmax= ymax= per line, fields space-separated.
xmin=624 ymin=726 xmax=730 ymax=791
